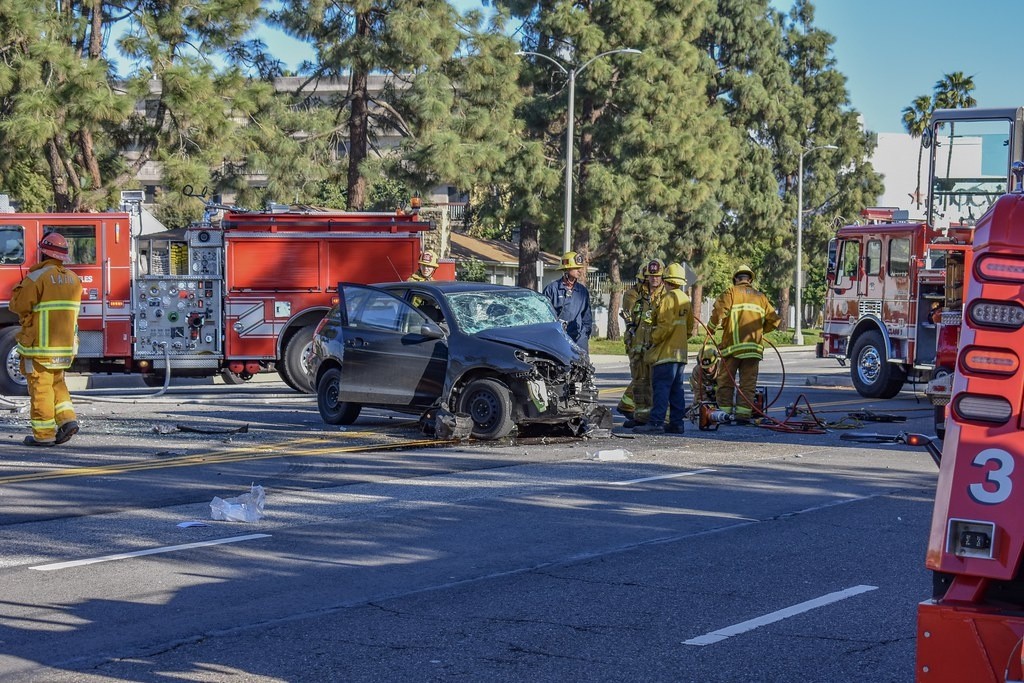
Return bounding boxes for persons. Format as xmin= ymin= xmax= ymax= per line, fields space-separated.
xmin=706 ymin=264 xmax=781 ymax=423
xmin=9 ymin=232 xmax=82 ymax=448
xmin=616 ymin=258 xmax=665 ymax=428
xmin=405 ymin=250 xmax=439 ymax=307
xmin=632 ymin=263 xmax=695 ymax=434
xmin=544 ymin=251 xmax=593 ymax=356
xmin=688 ymin=347 xmax=731 ymax=422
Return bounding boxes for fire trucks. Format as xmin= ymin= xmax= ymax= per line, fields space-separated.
xmin=814 ymin=109 xmax=1023 ymax=683
xmin=0 ymin=185 xmax=455 ymax=396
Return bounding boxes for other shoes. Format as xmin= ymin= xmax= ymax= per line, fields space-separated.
xmin=616 ymin=406 xmax=634 ymax=421
xmin=632 ymin=423 xmax=666 ymax=434
xmin=735 ymin=417 xmax=751 ymax=426
xmin=663 ymin=423 xmax=684 ymax=434
xmin=53 ymin=421 xmax=79 ymax=444
xmin=23 ymin=435 xmax=55 ymax=447
xmin=623 ymin=420 xmax=646 ymax=428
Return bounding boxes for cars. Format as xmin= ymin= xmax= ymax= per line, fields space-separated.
xmin=308 ymin=280 xmax=614 ymax=443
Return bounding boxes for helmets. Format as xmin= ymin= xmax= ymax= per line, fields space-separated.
xmin=661 ymin=262 xmax=688 ymax=286
xmin=644 ymin=258 xmax=666 ymax=276
xmin=732 ymin=264 xmax=755 ymax=284
xmin=697 ymin=344 xmax=719 ymax=369
xmin=37 ymin=232 xmax=71 ymax=261
xmin=636 ymin=262 xmax=651 ymax=281
xmin=417 ymin=249 xmax=439 ymax=268
xmin=555 ymin=252 xmax=584 ymax=270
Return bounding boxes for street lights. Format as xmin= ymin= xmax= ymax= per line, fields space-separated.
xmin=514 ymin=49 xmax=643 ymax=255
xmin=792 ymin=145 xmax=837 ymax=345
